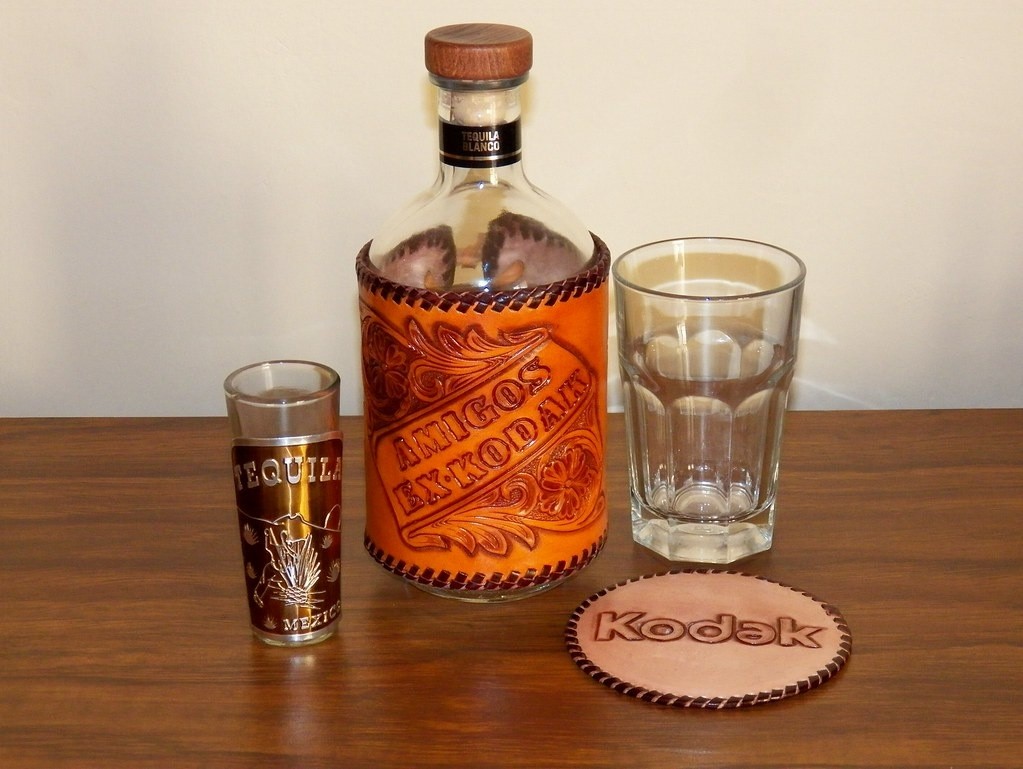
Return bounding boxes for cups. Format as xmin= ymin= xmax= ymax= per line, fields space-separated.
xmin=223 ymin=356 xmax=344 ymax=649
xmin=612 ymin=237 xmax=807 ymax=565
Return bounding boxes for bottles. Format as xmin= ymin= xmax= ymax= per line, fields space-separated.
xmin=356 ymin=20 xmax=611 ymax=602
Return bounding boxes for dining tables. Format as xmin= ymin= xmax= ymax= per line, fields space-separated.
xmin=0 ymin=407 xmax=1022 ymax=769
